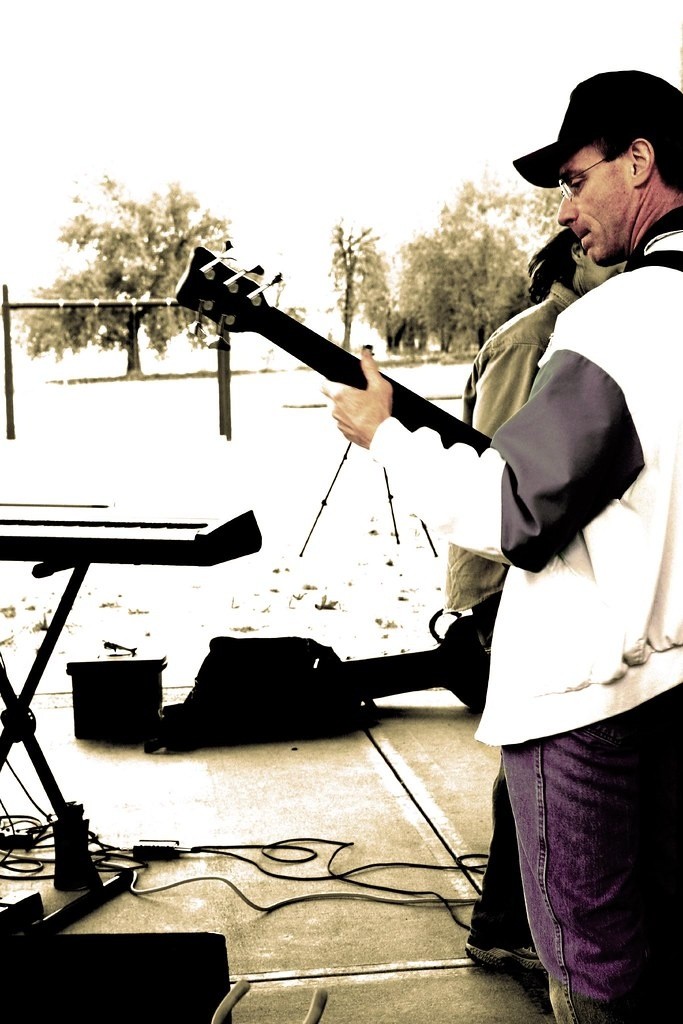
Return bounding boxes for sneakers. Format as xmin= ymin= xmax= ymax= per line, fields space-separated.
xmin=465 ymin=932 xmax=547 ymax=972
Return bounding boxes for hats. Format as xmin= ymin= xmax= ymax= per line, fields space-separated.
xmin=513 ymin=70 xmax=683 ymax=188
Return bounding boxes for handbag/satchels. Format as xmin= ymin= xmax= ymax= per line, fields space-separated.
xmin=143 ymin=637 xmax=407 ymax=755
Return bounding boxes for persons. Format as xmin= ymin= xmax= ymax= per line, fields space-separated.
xmin=450 ymin=226 xmax=628 ymax=975
xmin=324 ymin=72 xmax=683 ymax=1024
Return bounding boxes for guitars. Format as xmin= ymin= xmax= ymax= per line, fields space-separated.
xmin=174 ymin=240 xmax=491 ymax=458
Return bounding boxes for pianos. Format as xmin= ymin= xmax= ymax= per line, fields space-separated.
xmin=0 ymin=496 xmax=262 ymax=568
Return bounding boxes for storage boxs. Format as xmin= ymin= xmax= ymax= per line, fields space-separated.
xmin=66 ymin=655 xmax=168 ymax=749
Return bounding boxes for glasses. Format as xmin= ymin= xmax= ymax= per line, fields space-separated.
xmin=558 ymin=156 xmax=608 ymax=203
